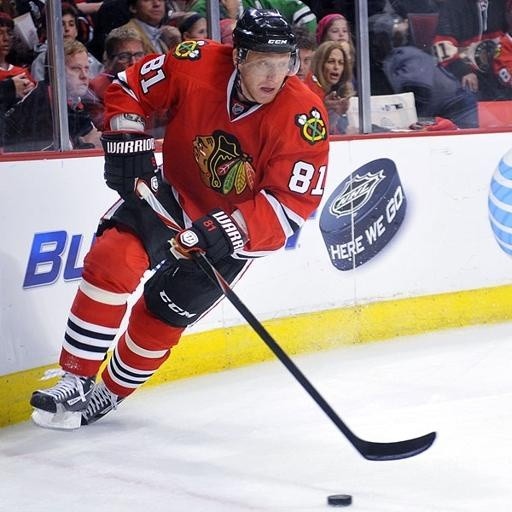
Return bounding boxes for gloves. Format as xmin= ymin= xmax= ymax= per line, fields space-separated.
xmin=97 ymin=131 xmax=158 ymax=204
xmin=165 ymin=206 xmax=245 ymax=274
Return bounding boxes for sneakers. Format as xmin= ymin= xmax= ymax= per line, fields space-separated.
xmin=30 ymin=375 xmax=97 ymax=411
xmin=83 ymin=381 xmax=124 ymax=425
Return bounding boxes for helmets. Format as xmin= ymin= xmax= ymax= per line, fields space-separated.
xmin=230 ymin=5 xmax=301 ymax=77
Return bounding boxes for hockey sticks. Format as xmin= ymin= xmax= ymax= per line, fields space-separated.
xmin=134 ymin=176 xmax=435 ymax=460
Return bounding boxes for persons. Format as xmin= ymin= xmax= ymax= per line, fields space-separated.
xmin=27 ymin=7 xmax=331 ymax=427
xmin=315 ymin=14 xmax=358 ymax=97
xmin=368 ymin=1 xmax=512 ymax=130
xmin=0 ymin=0 xmax=317 ymax=151
xmin=305 ymin=41 xmax=353 ymax=135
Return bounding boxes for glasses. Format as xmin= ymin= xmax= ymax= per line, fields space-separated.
xmin=109 ymin=53 xmax=145 ymax=66
